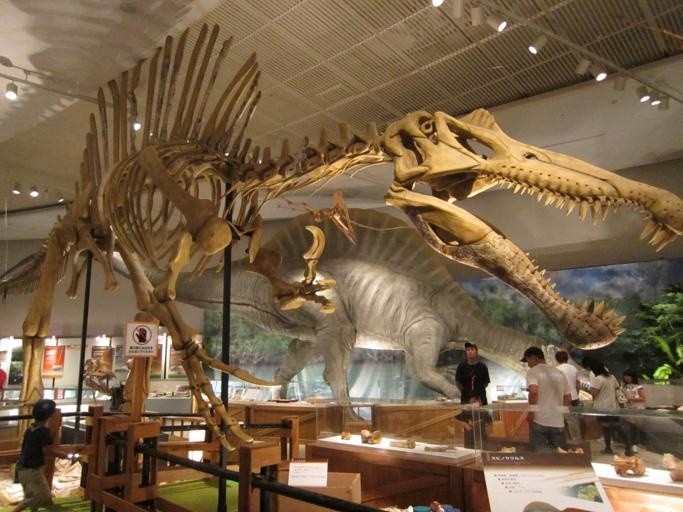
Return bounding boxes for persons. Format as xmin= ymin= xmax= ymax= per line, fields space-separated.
xmin=455 ymin=342 xmax=491 ymax=405
xmin=555 ymin=351 xmax=586 ymax=441
xmin=11 ymin=399 xmax=66 ymax=512
xmin=520 ymin=346 xmax=572 ymax=451
xmin=455 ymin=392 xmax=493 ymax=450
xmin=621 ymin=369 xmax=647 ymax=453
xmin=582 ymin=355 xmax=615 ymax=442
xmin=589 ymin=360 xmax=634 ymax=457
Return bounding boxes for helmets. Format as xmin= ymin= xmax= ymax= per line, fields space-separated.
xmin=32 ymin=400 xmax=55 ymax=421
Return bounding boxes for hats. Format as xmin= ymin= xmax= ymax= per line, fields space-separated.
xmin=465 ymin=342 xmax=477 ymax=349
xmin=520 ymin=347 xmax=544 ymax=362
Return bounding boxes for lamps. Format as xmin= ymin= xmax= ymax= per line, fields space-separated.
xmin=0 ymin=70 xmax=141 ymax=132
xmin=428 ymin=0 xmax=682 ymax=110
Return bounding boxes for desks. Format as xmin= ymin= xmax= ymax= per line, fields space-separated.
xmin=226 ymin=400 xmax=249 ymax=425
xmin=144 ymin=390 xmax=200 ymax=437
xmin=592 ymin=461 xmax=681 ymax=510
xmin=492 ymin=400 xmax=531 ymax=443
xmin=373 ymin=402 xmax=467 ymax=450
xmin=302 ymin=433 xmax=483 ymax=512
xmin=252 ymin=402 xmax=343 ymax=444
